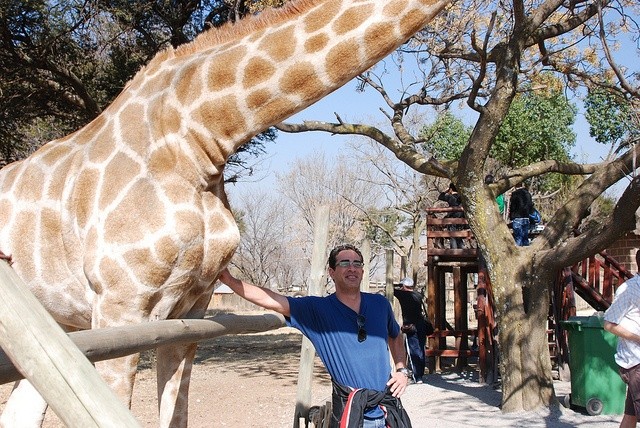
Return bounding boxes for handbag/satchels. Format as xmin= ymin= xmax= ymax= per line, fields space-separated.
xmin=423 ymin=318 xmax=434 ymax=337
xmin=400 ymin=323 xmax=415 ymax=334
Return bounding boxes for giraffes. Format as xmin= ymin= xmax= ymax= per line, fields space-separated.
xmin=0 ymin=0 xmax=453 ymax=427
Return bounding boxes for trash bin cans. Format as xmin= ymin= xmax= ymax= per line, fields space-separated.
xmin=560 ymin=316 xmax=628 ymax=416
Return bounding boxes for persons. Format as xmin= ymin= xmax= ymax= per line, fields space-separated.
xmin=509 ymin=181 xmax=533 ymax=246
xmin=394 ymin=278 xmax=428 ymax=384
xmin=438 ymin=182 xmax=467 ymax=249
xmin=219 ymin=244 xmax=413 ymax=428
xmin=603 ymin=250 xmax=640 ymax=427
xmin=484 ymin=175 xmax=504 ymax=219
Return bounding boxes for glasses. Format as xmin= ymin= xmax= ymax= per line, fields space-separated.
xmin=336 ymin=259 xmax=363 ymax=268
xmin=356 ymin=315 xmax=367 ymax=342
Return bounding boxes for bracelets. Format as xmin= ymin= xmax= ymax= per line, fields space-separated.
xmin=396 ymin=367 xmax=410 ymax=378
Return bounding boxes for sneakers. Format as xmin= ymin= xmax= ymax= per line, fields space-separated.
xmin=412 ymin=379 xmax=423 ymax=384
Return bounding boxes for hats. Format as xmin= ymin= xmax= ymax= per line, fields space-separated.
xmin=399 ymin=277 xmax=414 ymax=287
